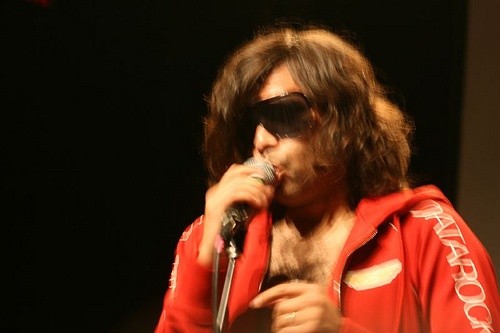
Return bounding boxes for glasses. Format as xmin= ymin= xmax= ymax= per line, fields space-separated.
xmin=231 ymin=91 xmax=316 ymax=152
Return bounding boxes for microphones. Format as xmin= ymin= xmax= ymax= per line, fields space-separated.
xmin=221 ymin=159 xmax=278 ymax=258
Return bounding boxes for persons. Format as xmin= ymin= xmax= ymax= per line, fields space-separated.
xmin=155 ymin=29 xmax=499 ymax=332
